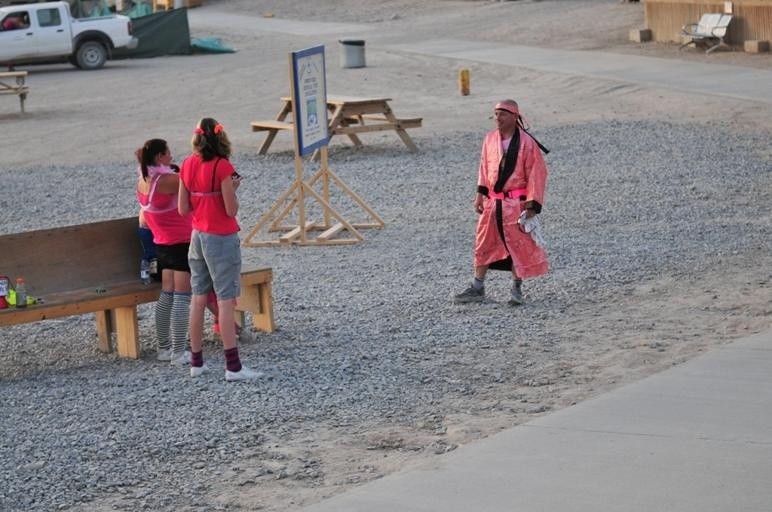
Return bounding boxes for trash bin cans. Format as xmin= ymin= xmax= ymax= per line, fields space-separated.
xmin=339 ymin=39 xmax=365 ymax=68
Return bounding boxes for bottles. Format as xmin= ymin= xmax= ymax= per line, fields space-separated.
xmin=139 ymin=255 xmax=152 ymax=286
xmin=15 ymin=278 xmax=28 ymax=310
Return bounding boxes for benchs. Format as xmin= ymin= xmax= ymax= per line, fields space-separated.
xmin=679 ymin=12 xmax=734 ymax=55
xmin=0 ymin=85 xmax=30 ymax=96
xmin=351 ymin=112 xmax=422 ymax=125
xmin=251 ymin=120 xmax=337 ymax=131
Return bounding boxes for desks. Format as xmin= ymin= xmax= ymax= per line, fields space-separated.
xmin=0 ymin=217 xmax=275 ymax=359
xmin=257 ymin=94 xmax=395 ymax=162
xmin=0 ymin=70 xmax=30 ymax=114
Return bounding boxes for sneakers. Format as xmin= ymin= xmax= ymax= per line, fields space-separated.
xmin=453 ymin=282 xmax=485 ymax=301
xmin=157 ymin=347 xmax=261 ymax=381
xmin=508 ymin=280 xmax=524 ymax=305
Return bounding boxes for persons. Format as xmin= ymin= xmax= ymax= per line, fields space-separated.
xmin=135 ymin=138 xmax=192 ymax=368
xmin=168 ymin=163 xmax=239 ymax=334
xmin=455 ymin=99 xmax=549 ymax=306
xmin=0 ymin=13 xmax=30 ymax=30
xmin=177 ymin=119 xmax=265 ymax=382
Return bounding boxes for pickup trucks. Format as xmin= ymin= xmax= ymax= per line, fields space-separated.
xmin=0 ymin=2 xmax=139 ymax=71
